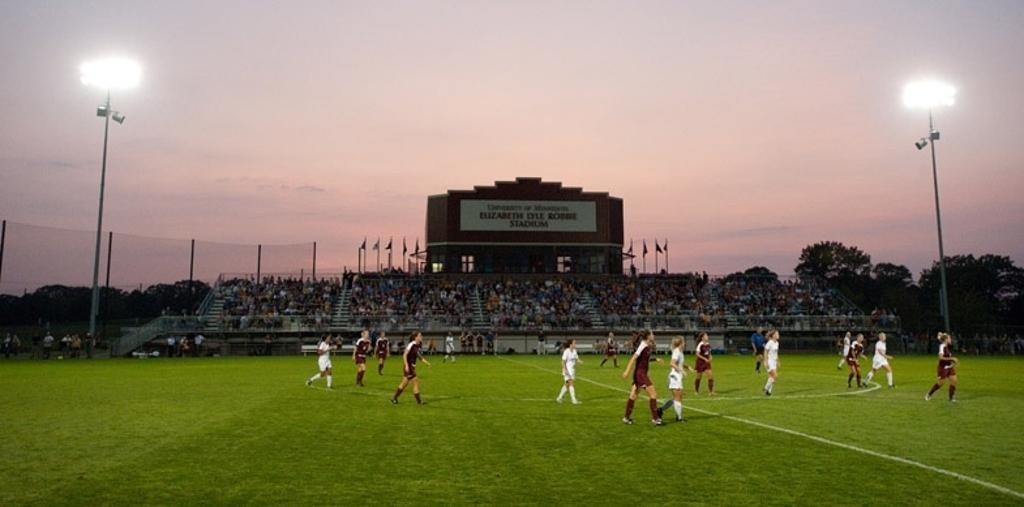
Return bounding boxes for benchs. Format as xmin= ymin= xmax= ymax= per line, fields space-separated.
xmin=195 ymin=268 xmax=903 ymax=339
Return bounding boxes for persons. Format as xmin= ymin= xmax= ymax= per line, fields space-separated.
xmin=3 ymin=325 xmax=94 ymax=362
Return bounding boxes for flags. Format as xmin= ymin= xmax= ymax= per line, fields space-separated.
xmin=628 ymin=244 xmax=667 ymax=255
xmin=362 ymin=240 xmax=419 ymax=252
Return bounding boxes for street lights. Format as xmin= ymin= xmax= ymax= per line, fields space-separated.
xmin=73 ymin=49 xmax=147 ymax=360
xmin=900 ymin=73 xmax=959 ymax=356
